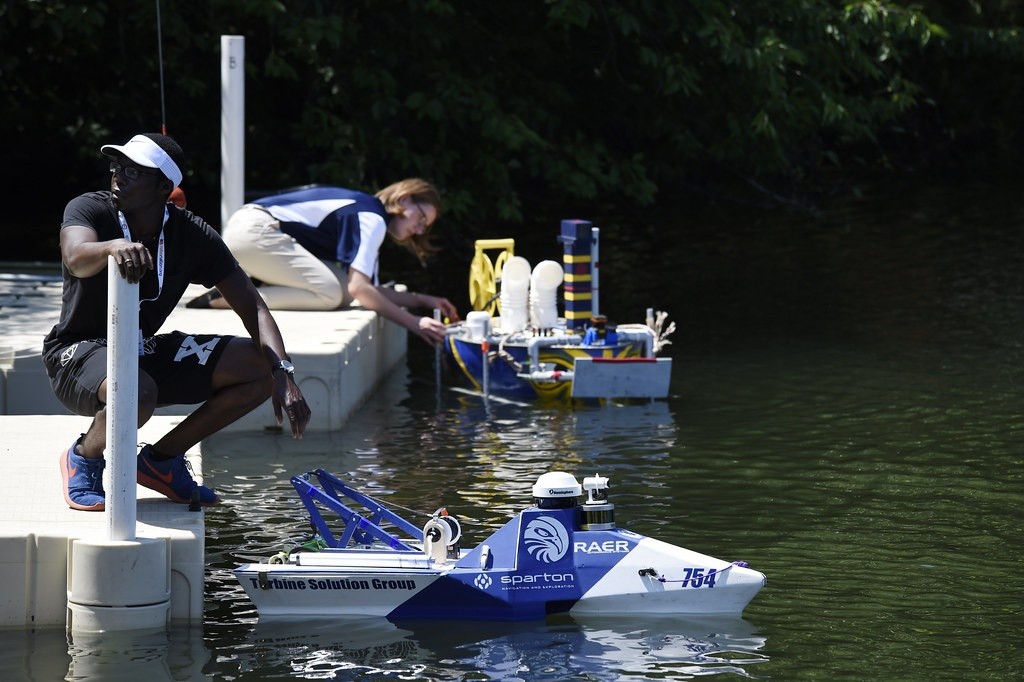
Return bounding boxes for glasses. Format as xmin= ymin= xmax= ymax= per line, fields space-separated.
xmin=416 ymin=203 xmax=426 ymax=235
xmin=109 ymin=160 xmax=161 ymax=181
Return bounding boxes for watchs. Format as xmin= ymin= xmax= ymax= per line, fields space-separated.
xmin=271 ymin=358 xmax=295 ymax=380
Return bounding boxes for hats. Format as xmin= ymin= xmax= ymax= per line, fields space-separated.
xmin=100 ymin=133 xmax=184 ymax=190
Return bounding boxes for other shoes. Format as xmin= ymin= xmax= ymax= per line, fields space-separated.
xmin=250 ymin=277 xmax=263 ymax=287
xmin=186 ymin=288 xmax=222 ymax=309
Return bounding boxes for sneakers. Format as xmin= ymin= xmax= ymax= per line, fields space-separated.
xmin=58 ymin=433 xmax=106 ymax=511
xmin=137 ymin=444 xmax=219 ymax=507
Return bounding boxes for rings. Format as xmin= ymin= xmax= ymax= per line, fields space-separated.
xmin=125 ymin=259 xmax=133 ymax=264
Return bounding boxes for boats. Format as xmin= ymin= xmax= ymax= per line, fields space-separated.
xmin=431 ymin=216 xmax=677 ymax=405
xmin=232 ymin=469 xmax=768 ymax=622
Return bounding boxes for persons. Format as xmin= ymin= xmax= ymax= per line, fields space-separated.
xmin=185 ymin=180 xmax=459 ymax=346
xmin=44 ymin=132 xmax=313 ymax=510
